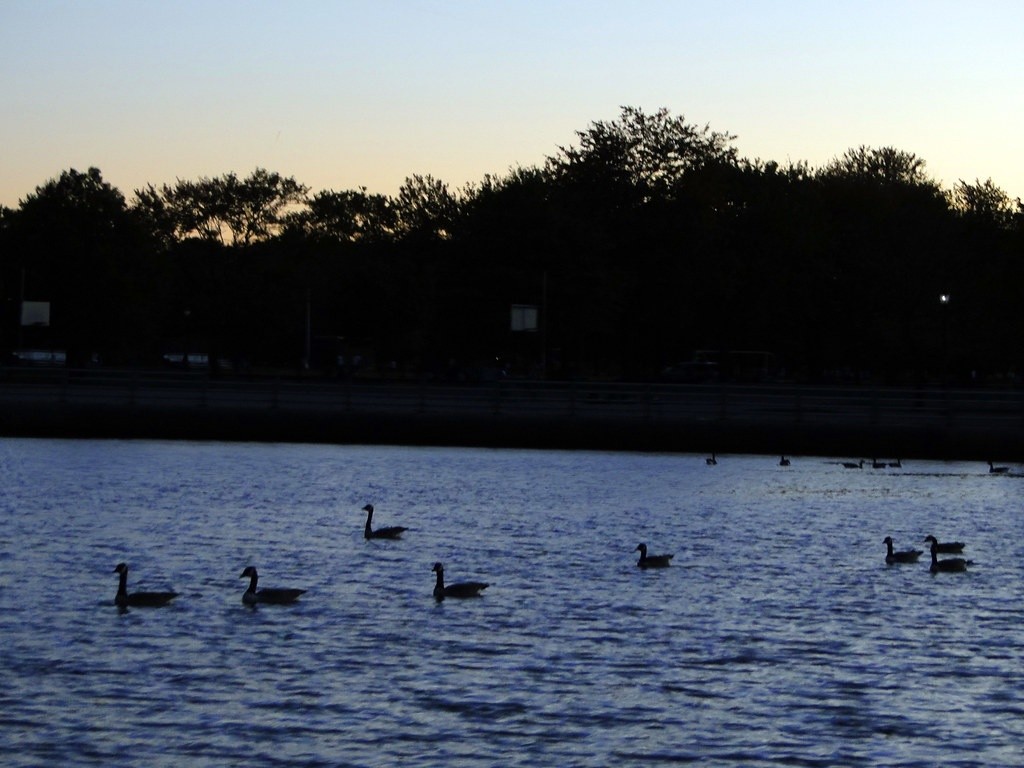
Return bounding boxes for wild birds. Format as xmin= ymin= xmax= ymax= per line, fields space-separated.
xmin=112 ymin=562 xmax=181 ymax=608
xmin=882 ymin=534 xmax=973 ymax=577
xmin=361 ymin=504 xmax=409 ymax=540
xmin=705 ymin=452 xmax=718 ymax=466
xmin=238 ymin=566 xmax=309 ymax=605
xmin=841 ymin=457 xmax=903 ymax=469
xmin=986 ymin=459 xmax=1010 ymax=473
xmin=431 ymin=562 xmax=490 ymax=600
xmin=634 ymin=542 xmax=675 ymax=568
xmin=779 ymin=453 xmax=791 ymax=466
